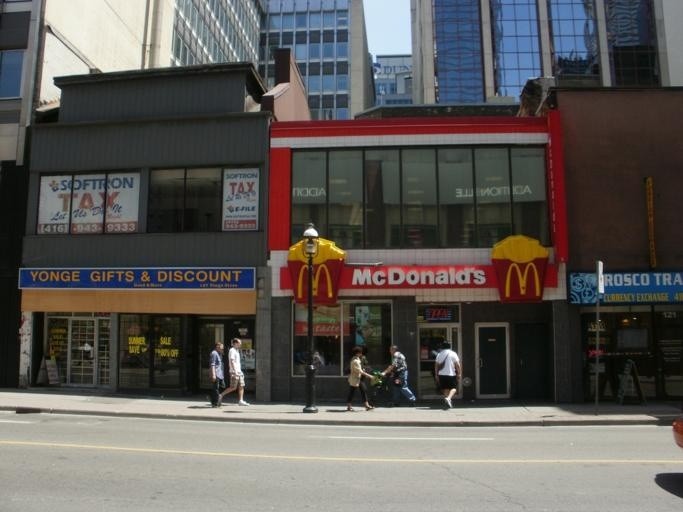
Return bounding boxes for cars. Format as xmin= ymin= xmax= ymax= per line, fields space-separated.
xmin=673 ymin=415 xmax=683 ymax=449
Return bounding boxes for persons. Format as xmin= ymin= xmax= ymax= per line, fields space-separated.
xmin=380 ymin=345 xmax=416 ymax=408
xmin=432 ymin=343 xmax=461 ymax=409
xmin=206 ymin=342 xmax=226 ymax=409
xmin=344 ymin=346 xmax=375 ymax=412
xmin=216 ymin=337 xmax=250 ymax=407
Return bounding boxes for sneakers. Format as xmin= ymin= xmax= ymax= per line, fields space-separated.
xmin=389 ymin=401 xmax=400 ymax=406
xmin=441 ymin=397 xmax=451 ymax=410
xmin=239 ymin=400 xmax=250 ymax=405
xmin=408 ymin=399 xmax=416 ymax=407
xmin=211 ymin=394 xmax=224 ymax=408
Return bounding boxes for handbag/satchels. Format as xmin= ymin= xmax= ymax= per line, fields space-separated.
xmin=394 ymin=377 xmax=402 ymax=387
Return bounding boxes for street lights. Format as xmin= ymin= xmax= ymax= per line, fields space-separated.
xmin=300 ymin=222 xmax=320 ymax=414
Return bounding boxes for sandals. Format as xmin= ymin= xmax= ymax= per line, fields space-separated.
xmin=347 ymin=408 xmax=354 ymax=412
xmin=364 ymin=405 xmax=374 ymax=411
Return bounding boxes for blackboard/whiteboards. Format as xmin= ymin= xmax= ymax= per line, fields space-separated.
xmin=617 ymin=362 xmax=633 ymax=399
